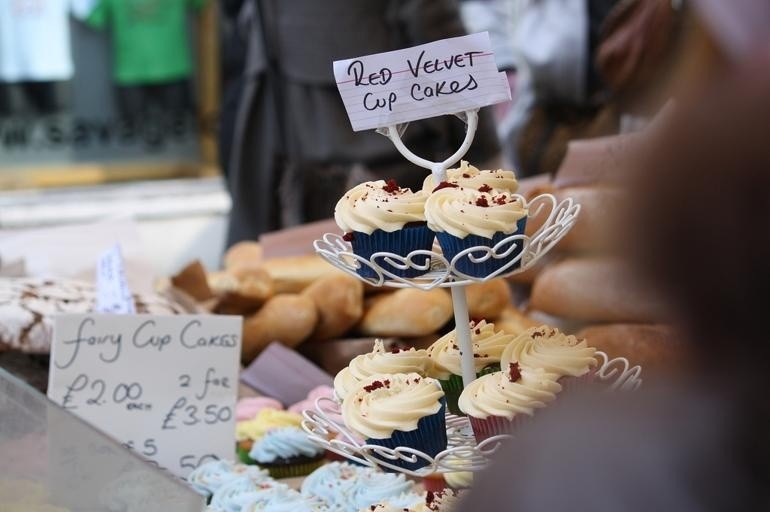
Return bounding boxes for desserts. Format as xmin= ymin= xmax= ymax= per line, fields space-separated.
xmin=187 ymin=320 xmax=597 ymax=512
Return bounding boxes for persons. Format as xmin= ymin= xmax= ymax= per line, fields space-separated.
xmin=77 ymin=0 xmax=210 ymax=137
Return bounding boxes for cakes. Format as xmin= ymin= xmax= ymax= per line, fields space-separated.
xmin=0 ymin=275 xmax=188 ymax=370
xmin=334 ymin=159 xmax=529 ymax=283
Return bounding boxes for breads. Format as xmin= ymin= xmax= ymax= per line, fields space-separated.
xmin=154 ymin=188 xmax=706 ymax=379
